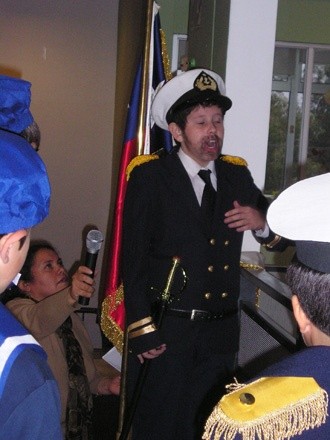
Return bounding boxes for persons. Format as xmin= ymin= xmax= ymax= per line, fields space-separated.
xmin=1 ymin=129 xmax=63 ymax=439
xmin=1 ymin=74 xmax=41 ymax=153
xmin=201 ymin=172 xmax=330 ymax=439
xmin=4 ymin=239 xmax=122 ymax=439
xmin=119 ymin=68 xmax=292 ymax=439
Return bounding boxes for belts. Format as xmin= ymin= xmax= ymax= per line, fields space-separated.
xmin=156 ymin=305 xmax=238 ymax=322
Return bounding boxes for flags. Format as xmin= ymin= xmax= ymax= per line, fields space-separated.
xmin=100 ymin=0 xmax=176 ymax=360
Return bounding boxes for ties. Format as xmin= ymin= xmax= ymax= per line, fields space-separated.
xmin=198 ymin=170 xmax=216 ymax=232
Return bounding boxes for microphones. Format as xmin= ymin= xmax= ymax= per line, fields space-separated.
xmin=78 ymin=230 xmax=103 ymax=305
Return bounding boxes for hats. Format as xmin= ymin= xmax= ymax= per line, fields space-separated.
xmin=0 ymin=75 xmax=33 ymax=134
xmin=0 ymin=129 xmax=51 ymax=235
xmin=151 ymin=68 xmax=232 ymax=132
xmin=266 ymin=172 xmax=330 ymax=274
